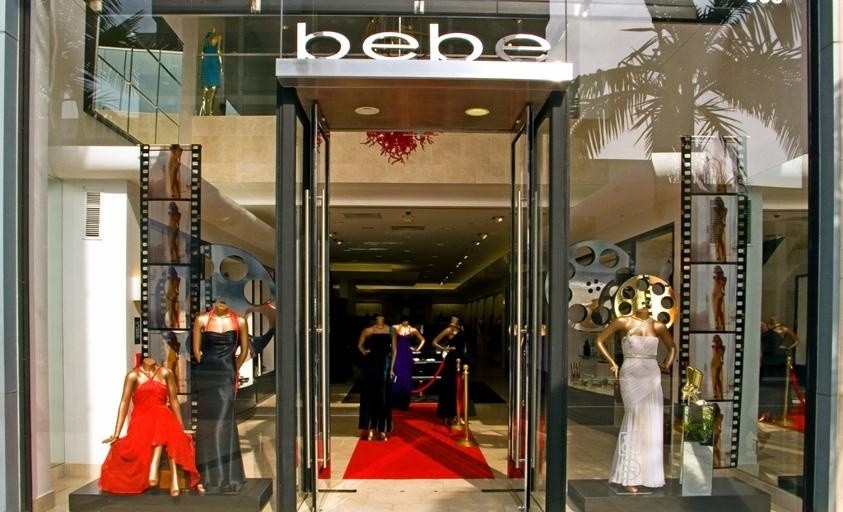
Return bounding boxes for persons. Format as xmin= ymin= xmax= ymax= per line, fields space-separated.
xmin=710 ymin=264 xmax=728 ymax=330
xmin=708 ymin=333 xmax=727 ymax=401
xmin=704 ymin=136 xmax=729 ymax=195
xmin=391 ymin=318 xmax=425 ymax=411
xmin=162 ymin=332 xmax=181 ymax=384
xmin=167 ymin=204 xmax=181 ymax=263
xmin=432 ymin=317 xmax=469 ymax=426
xmin=197 ymin=25 xmax=223 ymax=116
xmin=707 ymin=402 xmax=724 ymax=468
xmin=758 ymin=315 xmax=799 ymax=423
xmin=190 ymin=301 xmax=247 ymax=491
xmin=167 ymin=142 xmax=184 ymax=199
xmin=709 ymin=195 xmax=729 ymax=262
xmin=164 ymin=268 xmax=180 ymax=329
xmin=357 ymin=312 xmax=396 ymax=441
xmin=94 ymin=358 xmax=207 ymax=498
xmin=593 ymin=304 xmax=670 ymax=493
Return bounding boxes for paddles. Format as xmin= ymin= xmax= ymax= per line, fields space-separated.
xmin=786 ymin=405 xmax=804 ymax=434
xmin=342 ymin=402 xmax=494 ymax=480
